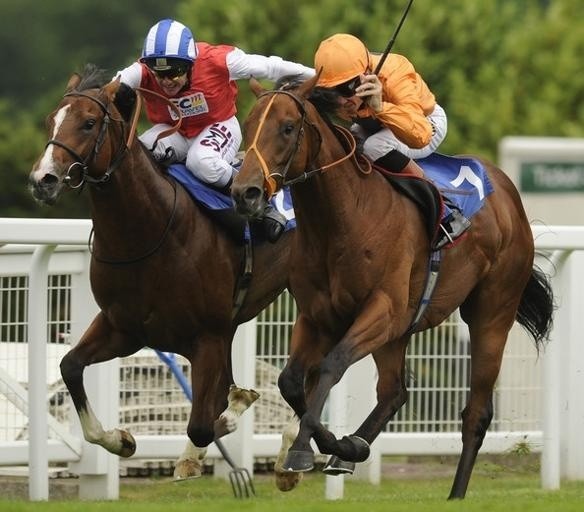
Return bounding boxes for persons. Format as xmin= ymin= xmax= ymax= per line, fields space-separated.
xmin=111 ymin=18 xmax=316 ymax=244
xmin=315 ymin=31 xmax=473 ymax=251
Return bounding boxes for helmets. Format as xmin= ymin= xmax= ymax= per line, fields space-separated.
xmin=138 ymin=19 xmax=198 ymax=63
xmin=313 ymin=33 xmax=371 ymax=88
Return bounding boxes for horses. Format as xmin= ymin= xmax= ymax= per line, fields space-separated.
xmin=221 ymin=65 xmax=555 ymax=504
xmin=25 ymin=63 xmax=326 ymax=492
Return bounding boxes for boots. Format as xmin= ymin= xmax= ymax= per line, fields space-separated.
xmin=219 ymin=166 xmax=286 ymax=244
xmin=398 ymin=157 xmax=471 ymax=251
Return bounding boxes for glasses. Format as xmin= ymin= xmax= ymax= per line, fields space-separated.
xmin=335 ymin=77 xmax=361 ymax=98
xmin=150 ymin=65 xmax=189 ymax=80
